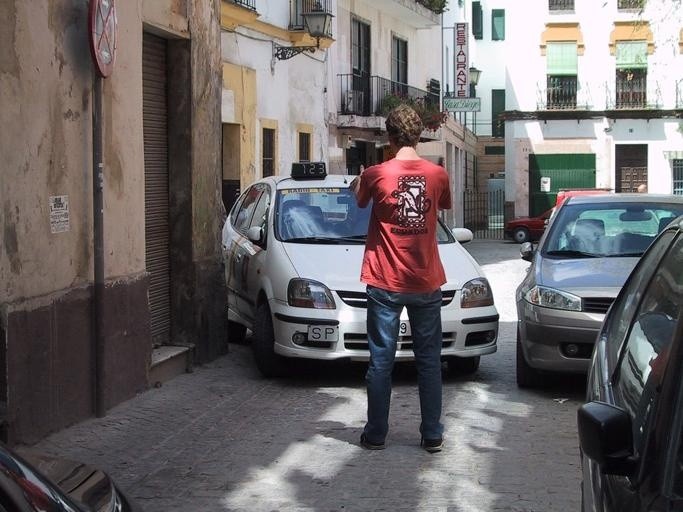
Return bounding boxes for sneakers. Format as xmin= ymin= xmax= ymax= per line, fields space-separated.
xmin=359 ymin=431 xmax=386 ymax=450
xmin=421 ymin=432 xmax=445 ymax=451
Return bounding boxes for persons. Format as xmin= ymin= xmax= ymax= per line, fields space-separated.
xmin=353 ymin=103 xmax=452 ymax=453
xmin=637 ymin=184 xmax=648 ymax=194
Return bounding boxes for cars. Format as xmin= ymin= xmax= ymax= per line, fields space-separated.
xmin=0 ymin=440 xmax=144 ymax=512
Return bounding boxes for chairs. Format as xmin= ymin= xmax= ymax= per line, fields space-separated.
xmin=280 ymin=200 xmax=316 ymax=239
xmin=574 ymin=219 xmax=606 ymax=252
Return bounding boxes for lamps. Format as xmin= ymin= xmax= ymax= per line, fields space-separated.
xmin=468 ymin=62 xmax=483 ymax=98
xmin=276 ymin=2 xmax=335 ymax=60
